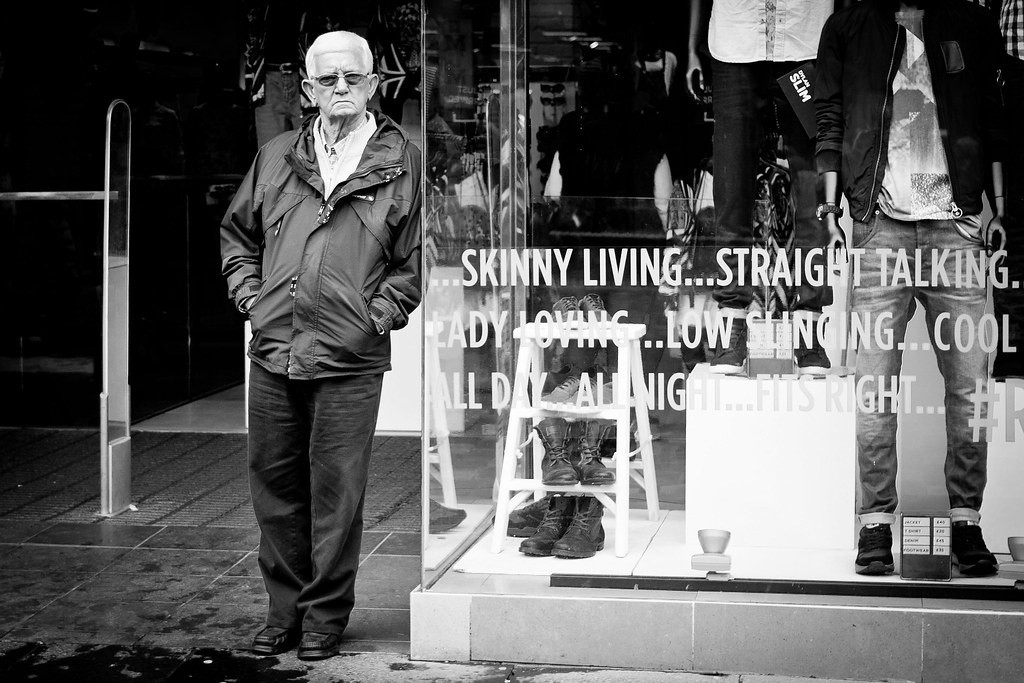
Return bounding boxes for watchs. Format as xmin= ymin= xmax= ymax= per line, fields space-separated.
xmin=815 ymin=203 xmax=843 ymax=221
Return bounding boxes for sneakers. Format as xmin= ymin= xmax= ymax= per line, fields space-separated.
xmin=542 ymin=378 xmax=615 ymax=412
xmin=792 ymin=325 xmax=831 ymax=375
xmin=951 ymin=524 xmax=1001 ymax=573
xmin=854 ymin=524 xmax=894 ymax=573
xmin=550 ymin=292 xmax=614 ymax=322
xmin=710 ymin=317 xmax=747 ymax=374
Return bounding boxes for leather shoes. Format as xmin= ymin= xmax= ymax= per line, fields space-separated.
xmin=252 ymin=625 xmax=295 ymax=654
xmin=298 ymin=631 xmax=339 ymax=658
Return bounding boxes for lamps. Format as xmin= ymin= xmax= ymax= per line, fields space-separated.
xmin=691 ymin=529 xmax=731 ymax=581
xmin=998 ymin=536 xmax=1024 ymax=590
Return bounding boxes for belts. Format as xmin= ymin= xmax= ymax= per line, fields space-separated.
xmin=264 ymin=63 xmax=302 ymax=75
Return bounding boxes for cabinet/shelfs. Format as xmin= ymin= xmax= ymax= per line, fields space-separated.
xmin=492 ymin=322 xmax=660 ymax=558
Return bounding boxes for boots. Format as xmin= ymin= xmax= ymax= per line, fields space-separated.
xmin=520 ymin=496 xmax=576 ymax=555
xmin=570 ymin=420 xmax=616 ymax=485
xmin=506 ymin=489 xmax=562 ymax=537
xmin=533 ymin=417 xmax=578 ymax=483
xmin=553 ymin=496 xmax=605 ymax=559
xmin=429 ymin=500 xmax=466 ymax=532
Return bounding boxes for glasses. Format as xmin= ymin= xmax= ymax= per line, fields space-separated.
xmin=540 ymin=96 xmax=566 ymax=107
xmin=309 ymin=72 xmax=370 ymax=87
xmin=539 ymin=83 xmax=564 ymax=93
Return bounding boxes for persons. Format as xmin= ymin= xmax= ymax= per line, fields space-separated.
xmin=221 ymin=31 xmax=435 ymax=659
xmin=810 ymin=0 xmax=1024 ymax=577
xmin=235 ymin=0 xmax=347 ymax=156
xmin=686 ymin=0 xmax=834 ymax=380
xmin=541 ymin=46 xmax=675 ymax=441
